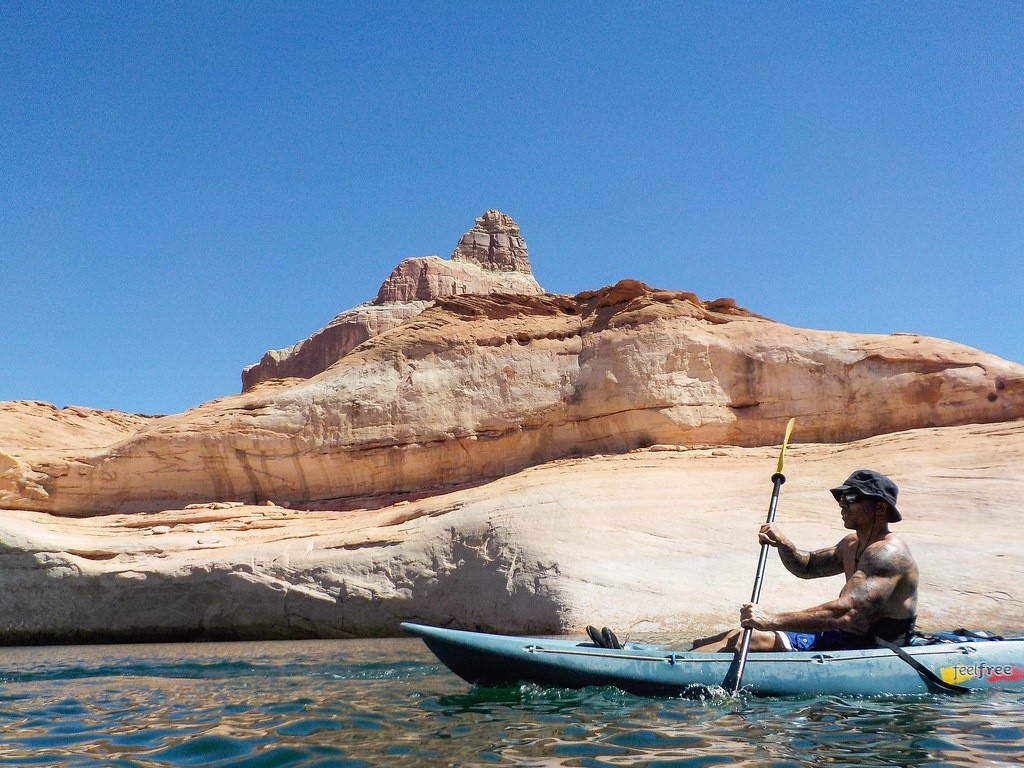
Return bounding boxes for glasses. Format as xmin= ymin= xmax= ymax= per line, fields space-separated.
xmin=840 ymin=492 xmax=869 ymax=503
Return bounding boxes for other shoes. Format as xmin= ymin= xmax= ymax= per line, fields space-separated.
xmin=586 ymin=626 xmax=605 ymax=648
xmin=601 ymin=627 xmax=630 ymax=649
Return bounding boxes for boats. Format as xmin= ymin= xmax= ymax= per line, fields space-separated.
xmin=399 ymin=623 xmax=1024 ymax=700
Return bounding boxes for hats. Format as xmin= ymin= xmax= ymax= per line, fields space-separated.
xmin=830 ymin=470 xmax=902 ymax=523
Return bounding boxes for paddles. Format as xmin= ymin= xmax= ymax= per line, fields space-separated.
xmin=726 ymin=414 xmax=797 ymax=701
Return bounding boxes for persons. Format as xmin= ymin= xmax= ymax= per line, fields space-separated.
xmin=586 ymin=470 xmax=919 ymax=649
